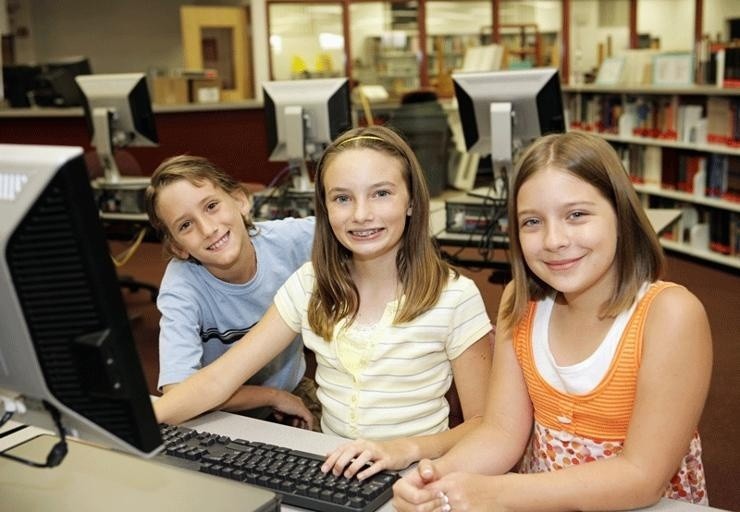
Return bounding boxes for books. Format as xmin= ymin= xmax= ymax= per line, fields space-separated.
xmin=582 ymin=96 xmax=740 ymax=256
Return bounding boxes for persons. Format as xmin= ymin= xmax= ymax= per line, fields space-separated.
xmin=153 ymin=124 xmax=494 ymax=482
xmin=145 ymin=154 xmax=321 ymax=432
xmin=393 ymin=130 xmax=714 ymax=512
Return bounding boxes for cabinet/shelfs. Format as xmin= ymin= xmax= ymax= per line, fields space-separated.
xmin=562 ymin=85 xmax=740 ymax=274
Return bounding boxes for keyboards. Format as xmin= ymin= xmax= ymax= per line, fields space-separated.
xmin=155 ymin=424 xmax=404 ymax=511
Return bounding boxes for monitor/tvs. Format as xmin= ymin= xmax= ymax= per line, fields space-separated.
xmin=451 ymin=68 xmax=566 ymax=200
xmin=74 ymin=73 xmax=164 ymax=186
xmin=0 ymin=141 xmax=163 ymax=461
xmin=262 ymin=78 xmax=352 ymax=194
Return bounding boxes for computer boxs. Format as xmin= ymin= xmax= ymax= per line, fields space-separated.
xmin=253 ymin=189 xmax=318 ymax=221
xmin=91 ymin=182 xmax=151 ymax=220
xmin=446 ymin=192 xmax=515 ymax=236
